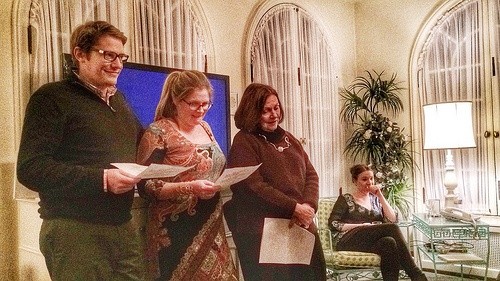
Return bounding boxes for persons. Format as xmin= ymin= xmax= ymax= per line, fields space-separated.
xmin=136 ymin=70 xmax=240 ymax=281
xmin=17 ymin=21 xmax=148 ymax=281
xmin=328 ymin=164 xmax=429 ymax=281
xmin=219 ymin=83 xmax=327 ymax=281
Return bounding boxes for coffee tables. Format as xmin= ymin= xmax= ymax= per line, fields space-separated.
xmin=414 ymin=213 xmax=490 ymax=281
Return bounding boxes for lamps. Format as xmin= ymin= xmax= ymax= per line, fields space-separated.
xmin=422 ymin=101 xmax=476 ymax=209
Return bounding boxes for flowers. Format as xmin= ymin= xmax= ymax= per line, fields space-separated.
xmin=339 ymin=70 xmax=411 ymax=218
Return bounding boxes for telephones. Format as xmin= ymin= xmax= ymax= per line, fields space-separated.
xmin=440 ymin=207 xmax=480 ymax=221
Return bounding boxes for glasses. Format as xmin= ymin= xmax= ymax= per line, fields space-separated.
xmin=173 ymin=91 xmax=213 ymax=111
xmin=90 ymin=48 xmax=129 ymax=63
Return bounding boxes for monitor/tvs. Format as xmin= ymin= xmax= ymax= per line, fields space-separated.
xmin=61 ymin=53 xmax=231 ymax=160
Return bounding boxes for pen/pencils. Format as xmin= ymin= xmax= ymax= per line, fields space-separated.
xmin=372 ymin=222 xmax=387 ymax=224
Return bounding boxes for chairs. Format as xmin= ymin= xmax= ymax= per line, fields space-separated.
xmin=316 ymin=197 xmax=385 ymax=281
